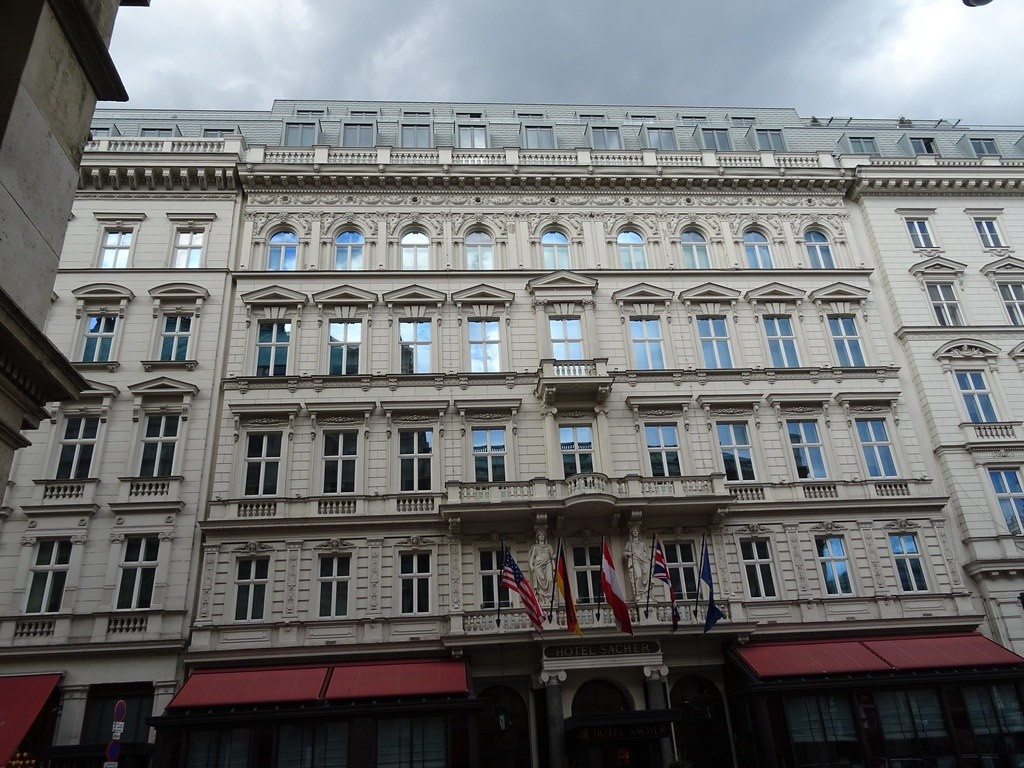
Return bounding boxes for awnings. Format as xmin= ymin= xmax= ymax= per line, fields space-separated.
xmin=166 ymin=660 xmax=469 ymax=710
xmin=0 ymin=672 xmax=63 ymax=768
xmin=736 ymin=633 xmax=1024 ymax=677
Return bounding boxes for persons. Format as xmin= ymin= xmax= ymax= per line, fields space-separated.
xmin=529 ymin=531 xmax=556 ymax=607
xmin=623 ymin=528 xmax=652 ymax=600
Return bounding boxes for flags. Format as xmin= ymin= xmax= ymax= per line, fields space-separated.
xmin=554 ymin=544 xmax=585 ymax=639
xmin=653 ymin=536 xmax=681 ymax=633
xmin=602 ymin=538 xmax=634 ymax=636
xmin=501 ymin=549 xmax=547 ymax=638
xmin=701 ymin=540 xmax=724 ymax=635
